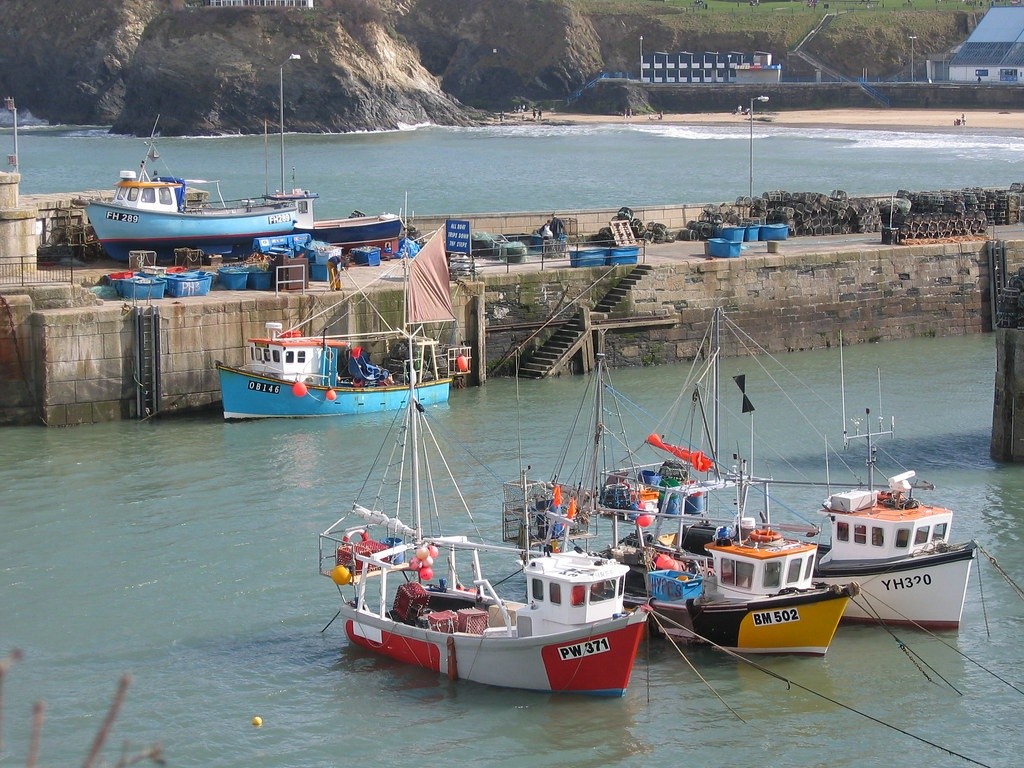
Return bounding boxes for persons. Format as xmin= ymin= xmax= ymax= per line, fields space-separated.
xmin=953 ymin=112 xmax=966 ymax=124
xmin=532 ymin=106 xmax=536 ymax=118
xmin=549 ymin=210 xmax=565 ymax=253
xmin=512 ymin=103 xmax=529 ymax=113
xmin=624 ymin=106 xmax=627 ymax=118
xmin=540 ymin=220 xmax=554 ymax=254
xmin=500 ymin=110 xmax=505 ymax=121
xmin=659 ymin=110 xmax=664 ymax=119
xmin=732 ymin=102 xmax=749 ymax=116
xmin=538 ymin=109 xmax=542 ymax=120
xmin=978 ymin=76 xmax=981 ymax=83
xmin=327 ymin=255 xmax=350 ymax=291
xmin=628 ymin=107 xmax=632 ymax=117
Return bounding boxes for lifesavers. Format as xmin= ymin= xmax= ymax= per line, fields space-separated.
xmin=343 ymin=529 xmax=368 ymax=547
xmin=750 ymin=528 xmax=782 ymax=541
xmin=282 ymin=330 xmax=301 ymax=337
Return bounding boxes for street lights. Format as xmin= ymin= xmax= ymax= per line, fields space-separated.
xmin=911 ymin=36 xmax=916 ymax=81
xmin=639 ymin=35 xmax=645 ymax=85
xmin=279 ymin=55 xmax=301 ymax=195
xmin=750 ymin=96 xmax=769 ymax=199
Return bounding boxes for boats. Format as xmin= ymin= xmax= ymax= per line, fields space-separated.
xmin=82 ymin=110 xmax=403 ymax=265
xmin=710 ymin=307 xmax=977 ymax=631
xmin=217 ymin=322 xmax=456 ymax=419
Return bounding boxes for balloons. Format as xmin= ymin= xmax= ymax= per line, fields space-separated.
xmin=408 ymin=545 xmax=438 ymax=580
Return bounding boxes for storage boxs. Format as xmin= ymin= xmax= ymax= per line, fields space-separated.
xmin=103 ymin=249 xmax=214 ymax=299
xmin=457 ymin=606 xmax=489 ymax=635
xmin=310 ymin=261 xmax=342 ymax=281
xmin=428 ymin=610 xmax=459 ymax=634
xmin=708 ymin=238 xmax=741 ymax=257
xmin=711 ymin=226 xmax=747 ymax=243
xmin=489 ymin=603 xmax=527 ymax=627
xmin=602 ymin=246 xmax=639 ymax=265
xmin=611 ymin=545 xmax=641 ymax=565
xmin=631 ymin=490 xmax=660 ymax=519
xmin=298 ymin=242 xmax=330 ymax=262
xmin=739 ymin=226 xmax=762 ymax=242
xmin=393 ymin=581 xmax=431 ymax=622
xmin=314 ymin=246 xmax=344 ymax=264
xmin=755 ymin=223 xmax=789 ymax=240
xmin=242 ymin=265 xmax=273 ymax=290
xmin=532 ymin=234 xmax=567 ymax=252
xmin=337 ymin=539 xmax=390 ymax=575
xmin=218 ymin=266 xmax=249 ymax=290
xmin=350 ymin=245 xmax=380 ymax=266
xmin=568 ymin=248 xmax=609 ymax=267
xmin=648 ymin=569 xmax=703 ymax=603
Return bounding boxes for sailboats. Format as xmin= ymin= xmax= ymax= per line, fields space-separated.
xmin=318 ymin=326 xmax=650 ymax=700
xmin=502 ymin=328 xmax=862 ymax=656
